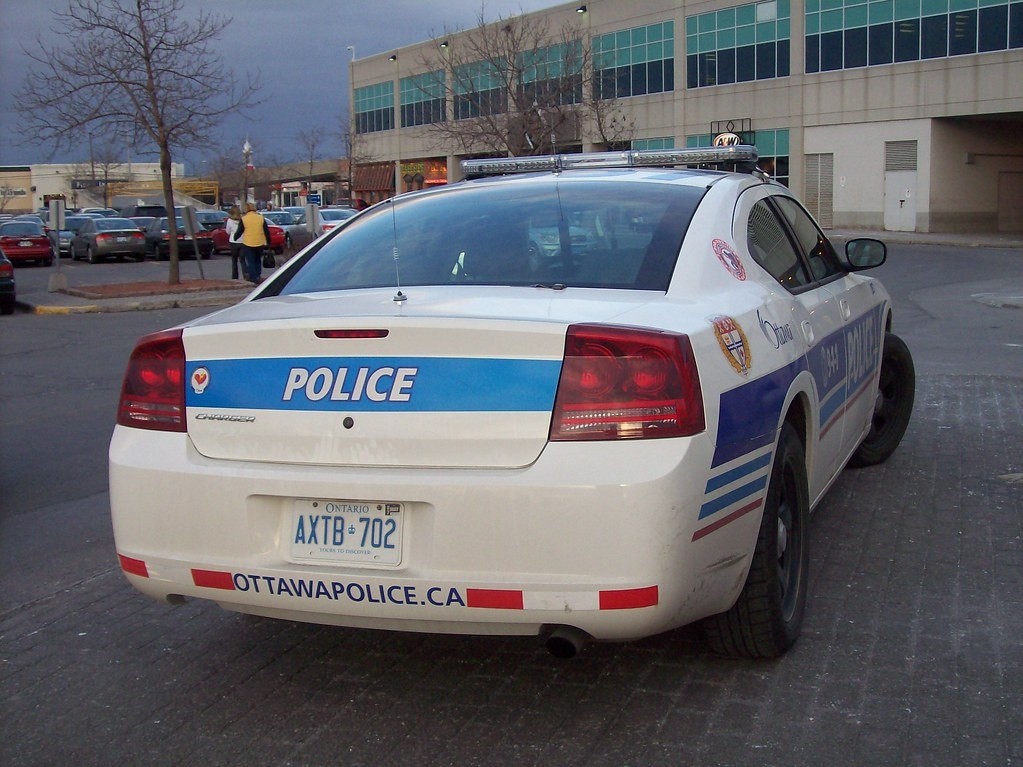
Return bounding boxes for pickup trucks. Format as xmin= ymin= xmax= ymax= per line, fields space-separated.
xmin=318 ymin=198 xmax=371 ymax=212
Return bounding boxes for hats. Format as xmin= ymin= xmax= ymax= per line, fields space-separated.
xmin=247 ymin=203 xmax=257 ymax=211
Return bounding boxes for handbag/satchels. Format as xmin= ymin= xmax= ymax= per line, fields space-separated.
xmin=263 ymin=246 xmax=276 ymax=269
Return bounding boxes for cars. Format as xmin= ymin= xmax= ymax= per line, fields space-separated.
xmin=45 ymin=216 xmax=89 ymax=253
xmin=140 ymin=217 xmax=213 ymax=259
xmin=70 ymin=218 xmax=147 ymax=262
xmin=0 ymin=248 xmax=18 ymax=314
xmin=0 ymin=222 xmax=55 ymax=267
xmin=256 ymin=206 xmax=306 ymax=226
xmin=527 ymin=212 xmax=598 ymax=271
xmin=212 ymin=216 xmax=285 ymax=255
xmin=108 ymin=142 xmax=916 ymax=662
xmin=1 ymin=207 xmax=231 ymax=227
xmin=285 ymin=209 xmax=358 ymax=248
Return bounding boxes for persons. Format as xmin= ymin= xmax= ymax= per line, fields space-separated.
xmin=264 ymin=203 xmax=273 ymax=212
xmin=226 ymin=202 xmax=271 ymax=284
